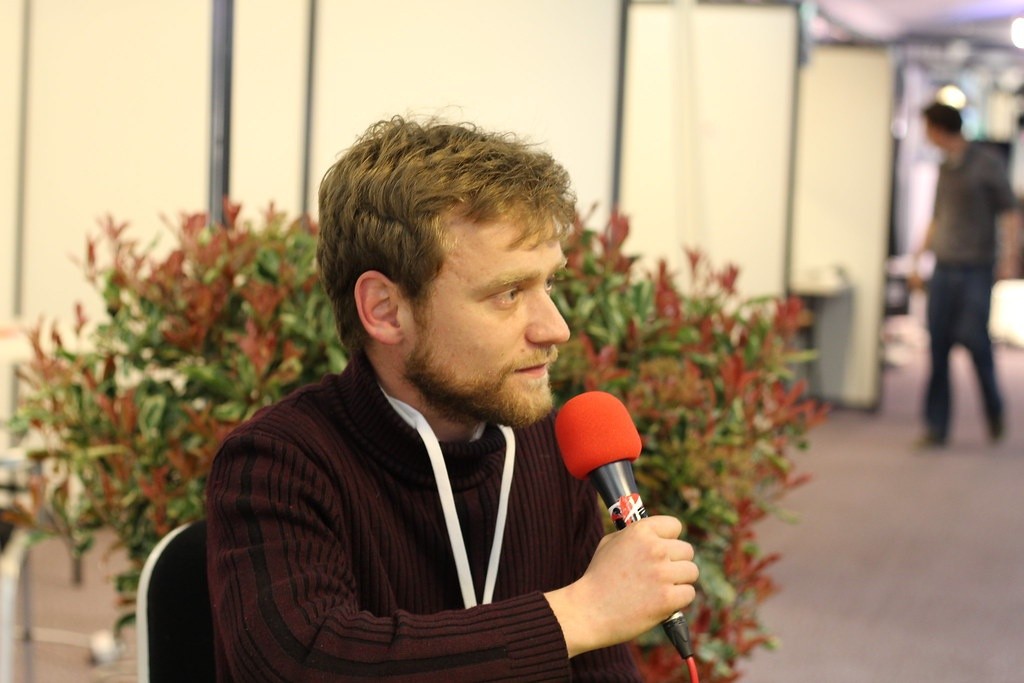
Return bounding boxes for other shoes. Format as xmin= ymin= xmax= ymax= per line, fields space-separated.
xmin=919 ymin=428 xmax=950 ymax=451
xmin=985 ymin=398 xmax=1003 ymax=440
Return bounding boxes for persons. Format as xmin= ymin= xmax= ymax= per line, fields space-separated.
xmin=203 ymin=115 xmax=700 ymax=683
xmin=905 ymin=102 xmax=1024 ymax=448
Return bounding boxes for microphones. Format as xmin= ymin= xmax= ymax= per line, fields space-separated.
xmin=552 ymin=390 xmax=693 ymax=659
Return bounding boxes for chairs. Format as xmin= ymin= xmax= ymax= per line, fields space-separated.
xmin=134 ymin=518 xmax=217 ymax=683
